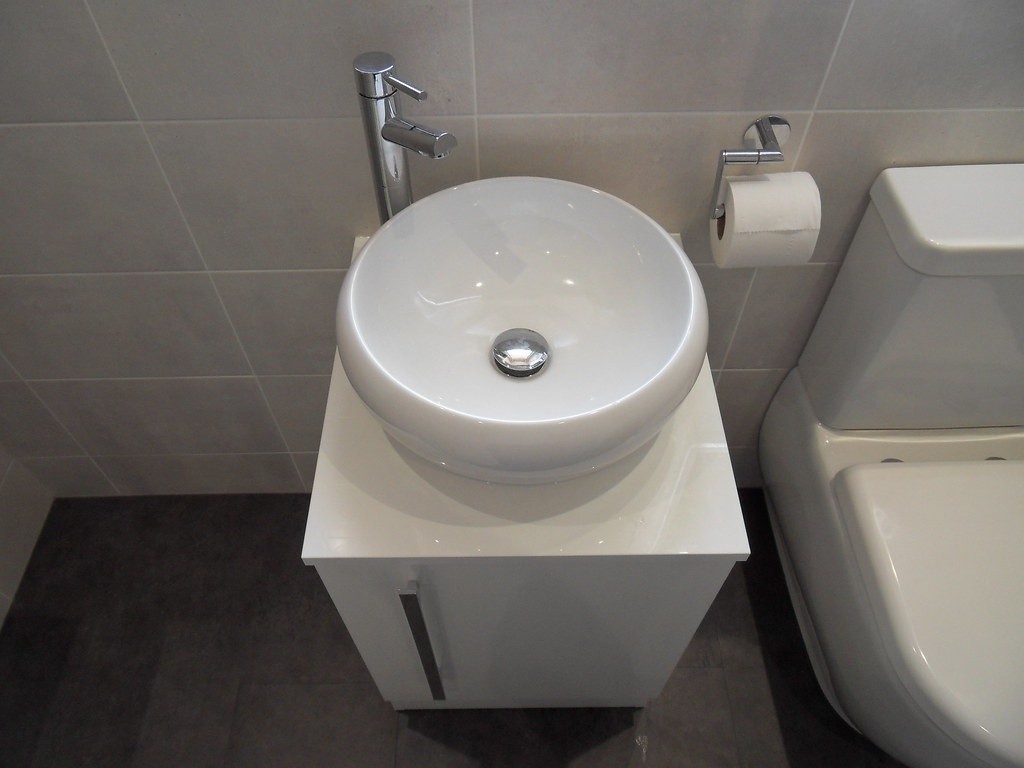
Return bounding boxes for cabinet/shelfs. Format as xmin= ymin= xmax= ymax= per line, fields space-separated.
xmin=299 ymin=234 xmax=751 ymax=709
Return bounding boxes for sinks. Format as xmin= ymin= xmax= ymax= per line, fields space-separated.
xmin=335 ymin=174 xmax=710 ymax=488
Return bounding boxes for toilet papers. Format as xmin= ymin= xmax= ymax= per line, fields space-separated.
xmin=709 ymin=170 xmax=823 ymax=270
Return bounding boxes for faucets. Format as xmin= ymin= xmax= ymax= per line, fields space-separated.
xmin=352 ymin=50 xmax=459 ymax=228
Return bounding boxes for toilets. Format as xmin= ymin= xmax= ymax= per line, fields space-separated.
xmin=755 ymin=162 xmax=1024 ymax=768
